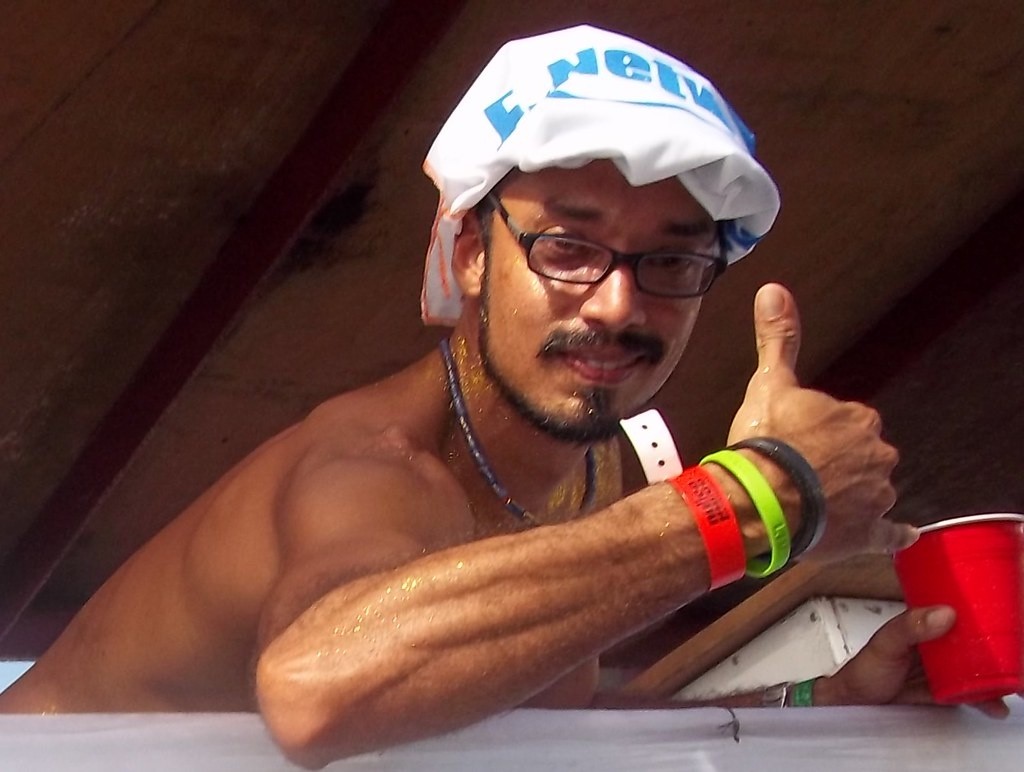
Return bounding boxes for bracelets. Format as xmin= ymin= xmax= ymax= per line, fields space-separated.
xmin=726 ymin=436 xmax=828 ymax=558
xmin=760 ymin=681 xmax=792 ymax=709
xmin=620 ymin=408 xmax=747 ymax=590
xmin=697 ymin=449 xmax=791 ymax=579
xmin=790 ymin=675 xmax=825 ymax=707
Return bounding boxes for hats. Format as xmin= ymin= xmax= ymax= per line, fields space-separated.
xmin=420 ymin=26 xmax=781 ymax=327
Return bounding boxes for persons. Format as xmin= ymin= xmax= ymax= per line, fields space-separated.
xmin=0 ymin=24 xmax=1024 ymax=772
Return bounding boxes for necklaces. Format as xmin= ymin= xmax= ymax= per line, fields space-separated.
xmin=439 ymin=336 xmax=597 ymax=528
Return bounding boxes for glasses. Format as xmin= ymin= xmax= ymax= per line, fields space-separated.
xmin=487 ymin=192 xmax=728 ymax=298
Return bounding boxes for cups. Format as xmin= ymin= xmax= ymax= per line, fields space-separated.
xmin=890 ymin=512 xmax=1023 ymax=708
